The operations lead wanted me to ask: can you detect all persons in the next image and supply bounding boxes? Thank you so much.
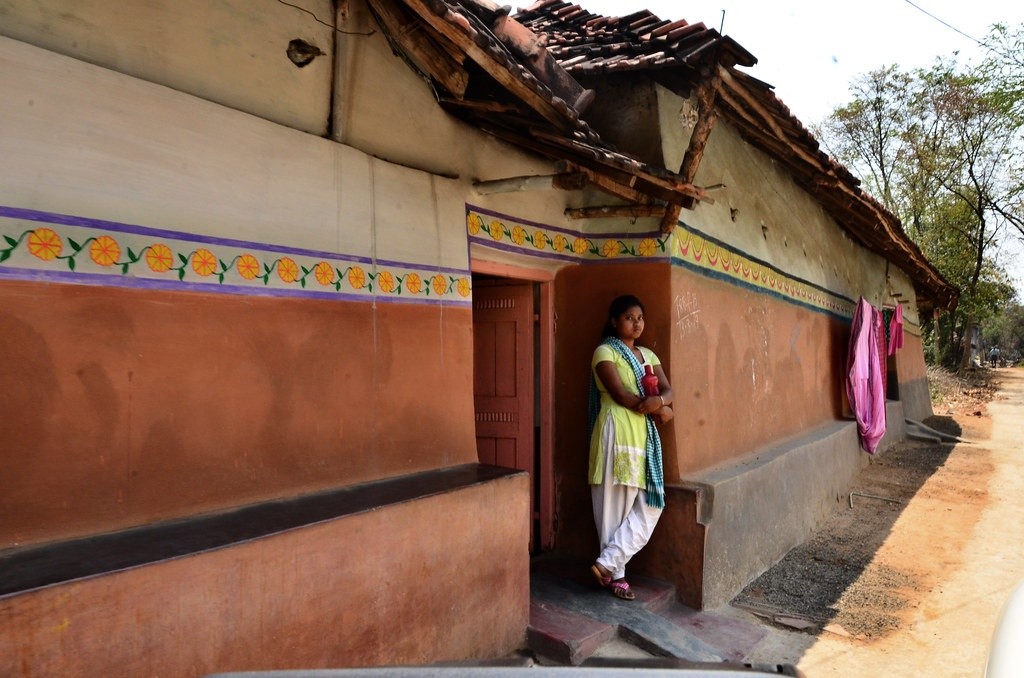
[588,293,675,600]
[987,347,1000,368]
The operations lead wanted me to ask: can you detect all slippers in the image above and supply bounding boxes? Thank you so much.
[612,582,636,600]
[591,565,610,588]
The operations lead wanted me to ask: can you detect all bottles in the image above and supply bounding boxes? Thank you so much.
[640,365,663,426]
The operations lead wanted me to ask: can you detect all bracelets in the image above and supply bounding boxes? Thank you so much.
[660,395,665,406]
